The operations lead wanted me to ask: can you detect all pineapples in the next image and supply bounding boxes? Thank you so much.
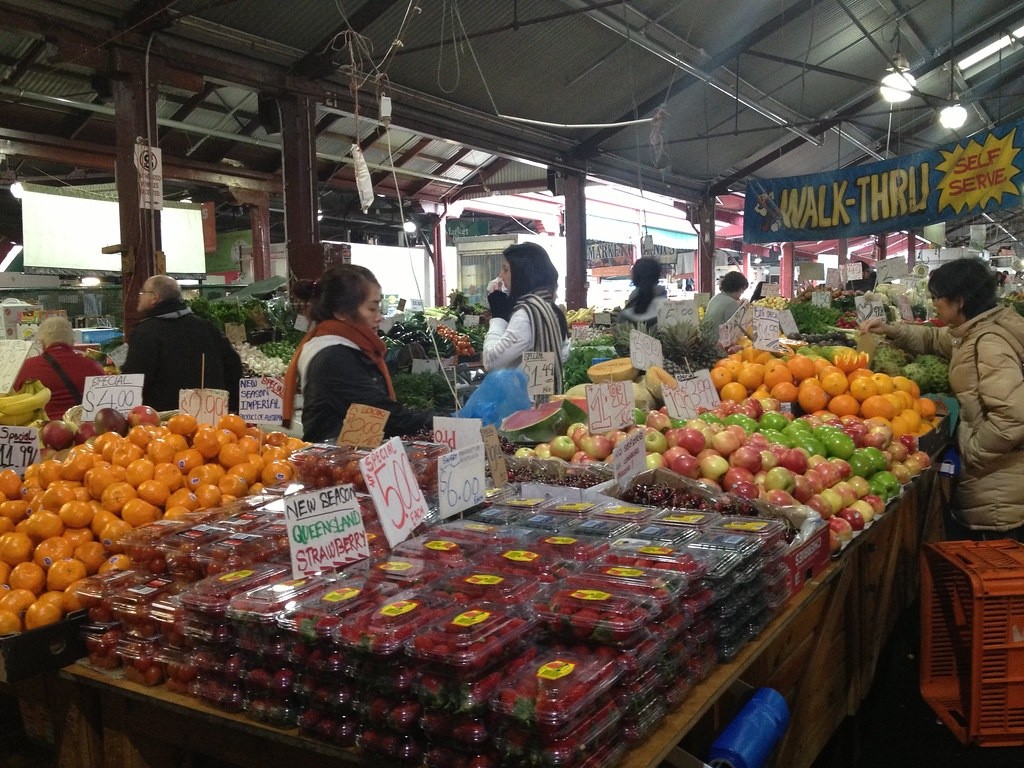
[611,321,728,377]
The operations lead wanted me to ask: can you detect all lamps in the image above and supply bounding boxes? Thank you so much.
[880,21,916,102]
[939,1,967,128]
[870,238,876,259]
[563,0,897,161]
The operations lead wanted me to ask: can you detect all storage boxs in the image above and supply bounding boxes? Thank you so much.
[920,539,1024,747]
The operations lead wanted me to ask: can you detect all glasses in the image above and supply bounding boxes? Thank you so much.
[139,289,153,295]
[931,296,953,302]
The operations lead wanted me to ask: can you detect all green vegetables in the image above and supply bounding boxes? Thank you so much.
[182,297,307,348]
[387,368,452,409]
[784,301,841,334]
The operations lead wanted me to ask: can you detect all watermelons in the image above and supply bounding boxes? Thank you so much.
[570,399,590,414]
[501,408,570,443]
[538,399,589,422]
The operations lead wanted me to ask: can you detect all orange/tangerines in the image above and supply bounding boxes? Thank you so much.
[0,413,314,634]
[708,345,943,440]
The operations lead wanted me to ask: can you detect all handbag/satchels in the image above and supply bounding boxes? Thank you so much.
[449,369,532,429]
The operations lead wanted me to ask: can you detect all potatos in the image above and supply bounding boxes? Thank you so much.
[788,285,864,306]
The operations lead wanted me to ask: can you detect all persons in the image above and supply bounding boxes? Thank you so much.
[858,255,1024,543]
[120,274,243,416]
[1014,260,1024,278]
[622,255,668,315]
[749,281,767,304]
[997,270,1011,287]
[13,317,106,421]
[297,263,433,443]
[701,270,748,327]
[841,260,874,295]
[482,240,568,395]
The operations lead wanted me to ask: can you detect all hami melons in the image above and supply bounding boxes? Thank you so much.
[567,356,679,411]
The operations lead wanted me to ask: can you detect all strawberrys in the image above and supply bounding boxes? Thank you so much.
[74,451,714,768]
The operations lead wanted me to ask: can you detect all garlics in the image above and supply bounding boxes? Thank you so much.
[231,341,289,376]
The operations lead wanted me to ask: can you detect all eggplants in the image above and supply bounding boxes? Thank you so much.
[377,324,432,350]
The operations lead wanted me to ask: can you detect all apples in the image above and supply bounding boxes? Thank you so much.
[512,398,929,553]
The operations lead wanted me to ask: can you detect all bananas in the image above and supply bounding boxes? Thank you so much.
[0,378,77,448]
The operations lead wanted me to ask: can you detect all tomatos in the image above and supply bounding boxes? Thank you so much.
[436,325,475,356]
